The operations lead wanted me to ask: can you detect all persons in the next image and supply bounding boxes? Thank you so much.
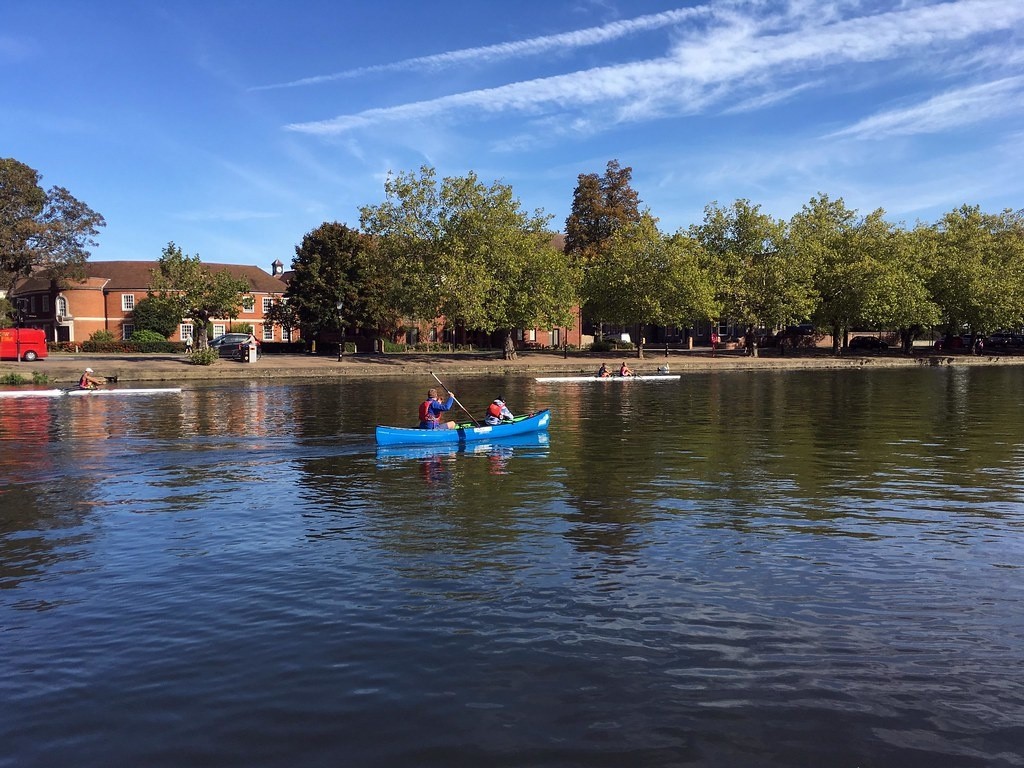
[185,333,193,354]
[598,363,609,377]
[242,332,255,354]
[486,395,514,426]
[418,389,456,430]
[79,368,98,388]
[620,362,632,376]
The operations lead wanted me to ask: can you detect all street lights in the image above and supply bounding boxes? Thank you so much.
[335,299,344,361]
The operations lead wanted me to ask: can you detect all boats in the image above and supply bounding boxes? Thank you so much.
[2,388,182,395]
[375,430,550,469]
[534,375,681,382]
[375,408,550,447]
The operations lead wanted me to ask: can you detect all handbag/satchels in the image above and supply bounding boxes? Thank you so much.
[185,341,186,345]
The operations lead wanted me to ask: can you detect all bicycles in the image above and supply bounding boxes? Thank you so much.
[232,343,262,362]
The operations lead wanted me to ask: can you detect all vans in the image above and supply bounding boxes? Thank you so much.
[0,325,49,360]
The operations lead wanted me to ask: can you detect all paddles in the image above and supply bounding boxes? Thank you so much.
[430,370,483,427]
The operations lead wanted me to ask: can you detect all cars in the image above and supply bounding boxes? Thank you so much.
[849,335,888,349]
[934,334,1024,349]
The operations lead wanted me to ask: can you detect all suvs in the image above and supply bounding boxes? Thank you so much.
[208,334,261,358]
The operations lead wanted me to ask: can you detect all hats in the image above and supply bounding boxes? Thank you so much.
[622,362,626,365]
[248,331,251,333]
[85,368,93,372]
[498,396,506,401]
[602,363,606,365]
